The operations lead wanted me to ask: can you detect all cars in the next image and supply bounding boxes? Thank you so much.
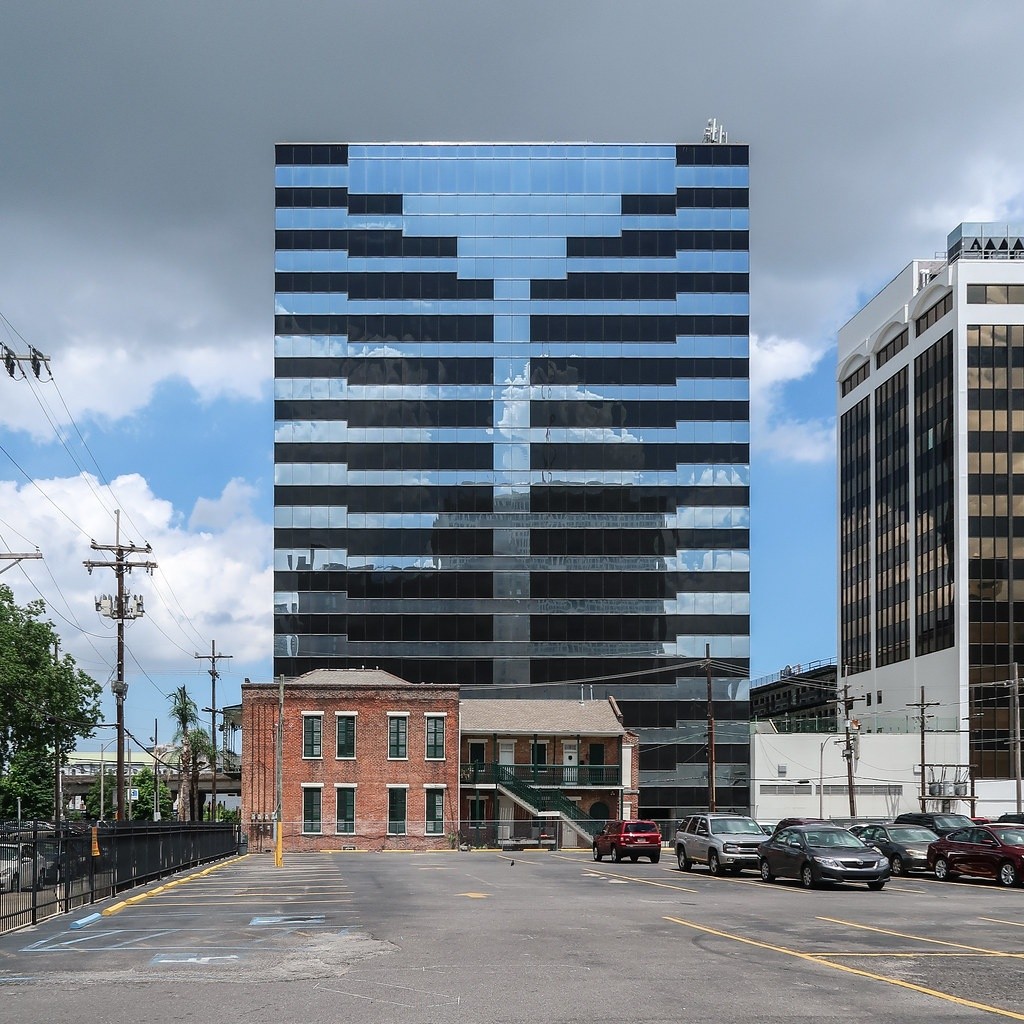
[755,824,892,891]
[855,823,941,876]
[0,819,112,894]
[926,822,1024,887]
[749,821,776,837]
[969,811,1024,827]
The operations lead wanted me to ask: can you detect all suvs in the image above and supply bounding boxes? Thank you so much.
[772,817,845,844]
[674,811,772,877]
[592,818,663,864]
[894,812,978,838]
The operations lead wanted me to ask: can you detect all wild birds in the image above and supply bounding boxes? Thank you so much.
[511,860,514,866]
